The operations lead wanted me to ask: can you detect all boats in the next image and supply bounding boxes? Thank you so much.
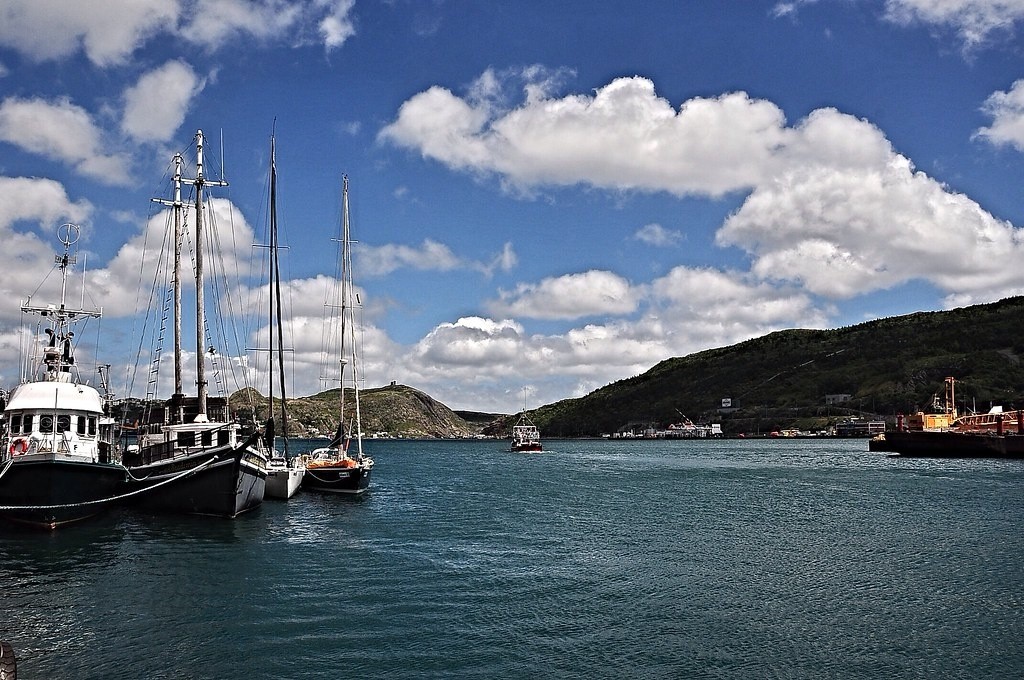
[510,413,542,453]
[0,220,124,527]
[884,377,1024,456]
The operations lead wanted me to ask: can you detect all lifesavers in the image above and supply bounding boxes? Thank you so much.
[10,439,27,457]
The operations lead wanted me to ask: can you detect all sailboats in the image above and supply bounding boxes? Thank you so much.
[128,131,266,519]
[306,173,374,494]
[247,117,307,498]
